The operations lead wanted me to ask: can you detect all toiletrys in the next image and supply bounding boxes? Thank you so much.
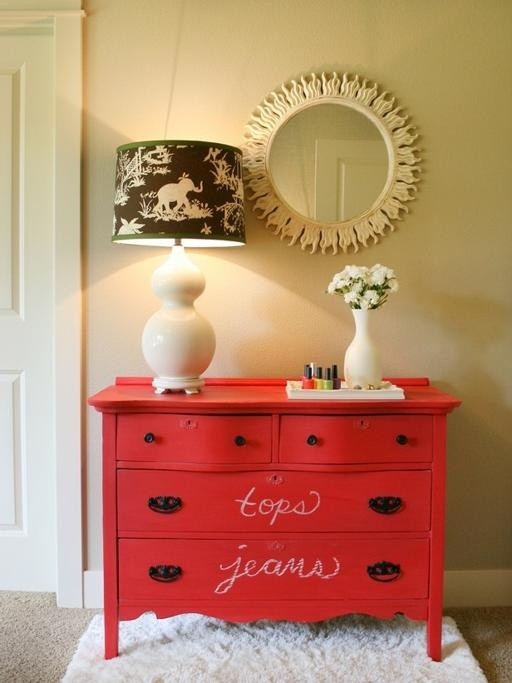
[300,359,343,391]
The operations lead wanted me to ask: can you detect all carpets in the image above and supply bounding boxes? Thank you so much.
[60,610,488,683]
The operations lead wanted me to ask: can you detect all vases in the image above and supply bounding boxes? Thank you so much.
[344,308,379,391]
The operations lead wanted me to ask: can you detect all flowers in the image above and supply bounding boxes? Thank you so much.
[325,262,398,310]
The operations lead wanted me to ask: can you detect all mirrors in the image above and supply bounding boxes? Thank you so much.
[240,71,422,256]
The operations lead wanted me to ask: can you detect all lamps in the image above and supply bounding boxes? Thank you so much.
[111,140,248,394]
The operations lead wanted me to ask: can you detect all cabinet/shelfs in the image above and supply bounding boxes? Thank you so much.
[102,409,447,662]
